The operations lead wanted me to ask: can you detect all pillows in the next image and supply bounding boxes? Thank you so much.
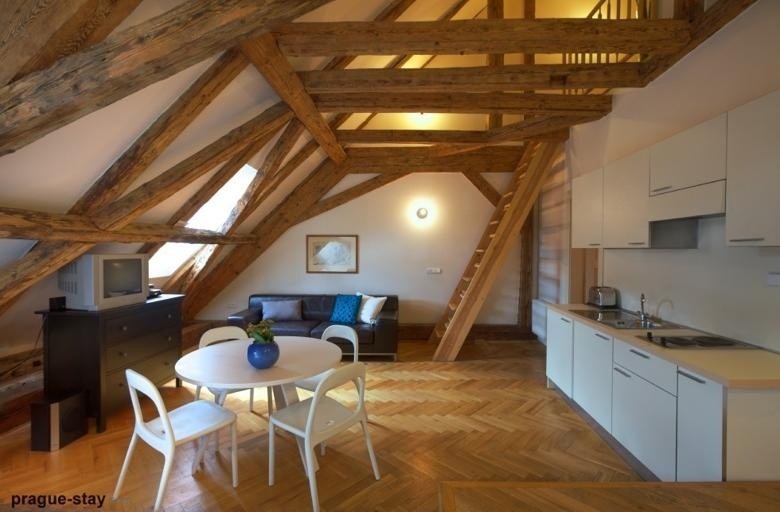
[356,292,386,323]
[261,300,302,320]
[331,293,362,324]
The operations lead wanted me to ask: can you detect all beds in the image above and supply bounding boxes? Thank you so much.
[174,336,342,473]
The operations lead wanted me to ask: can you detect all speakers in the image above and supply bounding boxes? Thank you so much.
[30,388,88,452]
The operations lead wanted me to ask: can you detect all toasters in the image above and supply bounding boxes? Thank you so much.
[588,285,618,309]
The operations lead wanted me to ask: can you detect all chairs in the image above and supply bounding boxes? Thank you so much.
[268,363,381,512]
[295,325,368,456]
[112,368,238,512]
[194,326,272,452]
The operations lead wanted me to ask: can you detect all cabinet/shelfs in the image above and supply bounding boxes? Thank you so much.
[570,88,780,250]
[546,307,779,483]
[34,293,187,433]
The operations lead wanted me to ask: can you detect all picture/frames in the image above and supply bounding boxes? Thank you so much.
[306,234,359,274]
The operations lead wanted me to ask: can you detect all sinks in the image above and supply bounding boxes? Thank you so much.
[598,319,663,331]
[567,306,639,320]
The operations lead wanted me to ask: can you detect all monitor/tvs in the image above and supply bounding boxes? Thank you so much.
[58,254,149,312]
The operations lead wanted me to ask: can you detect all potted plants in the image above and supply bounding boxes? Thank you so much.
[247,319,280,368]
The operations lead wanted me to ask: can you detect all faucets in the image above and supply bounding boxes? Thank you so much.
[640,293,648,327]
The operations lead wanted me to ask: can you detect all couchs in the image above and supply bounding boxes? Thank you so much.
[227,294,400,362]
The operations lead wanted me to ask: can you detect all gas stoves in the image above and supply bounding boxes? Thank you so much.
[634,332,763,349]
